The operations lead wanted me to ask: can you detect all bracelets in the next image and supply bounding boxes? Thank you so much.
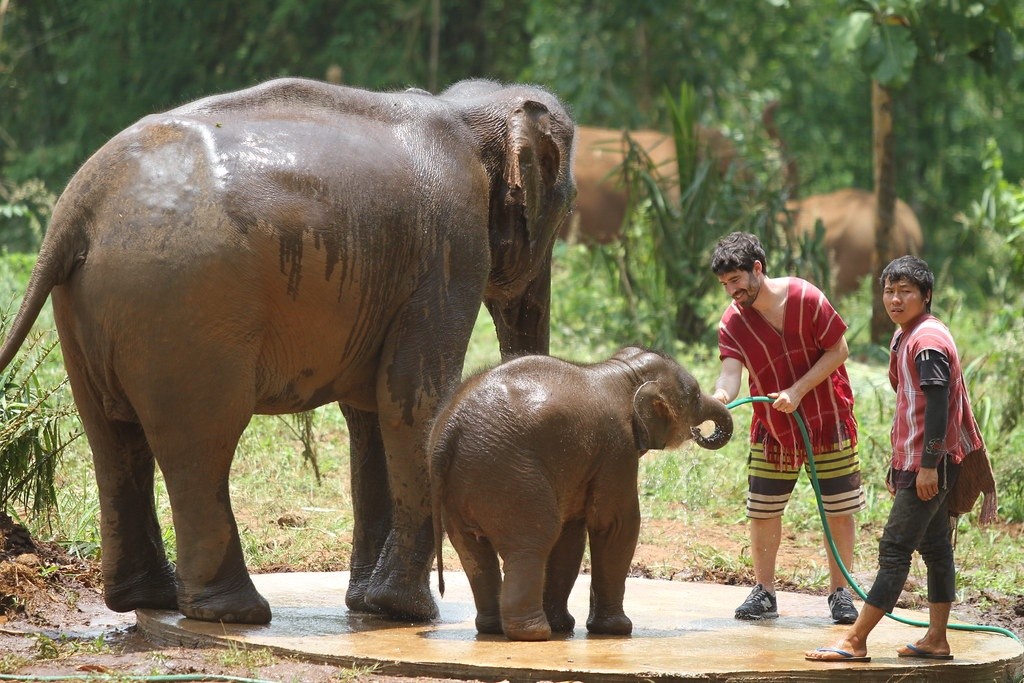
[715,389,728,406]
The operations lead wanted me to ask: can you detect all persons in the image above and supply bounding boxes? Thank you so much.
[803,254,980,662]
[712,231,863,624]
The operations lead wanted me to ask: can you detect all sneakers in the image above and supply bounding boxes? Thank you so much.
[735,583,779,619]
[828,587,859,622]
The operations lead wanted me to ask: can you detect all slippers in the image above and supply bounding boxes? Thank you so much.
[805,645,870,662]
[899,643,955,660]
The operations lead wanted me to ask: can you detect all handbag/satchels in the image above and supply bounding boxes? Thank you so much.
[884,415,998,526]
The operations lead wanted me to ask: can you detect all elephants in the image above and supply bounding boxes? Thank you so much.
[422,344,733,642]
[0,77,578,625]
[554,101,924,309]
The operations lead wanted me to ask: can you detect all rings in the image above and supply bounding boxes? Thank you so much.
[785,408,788,414]
